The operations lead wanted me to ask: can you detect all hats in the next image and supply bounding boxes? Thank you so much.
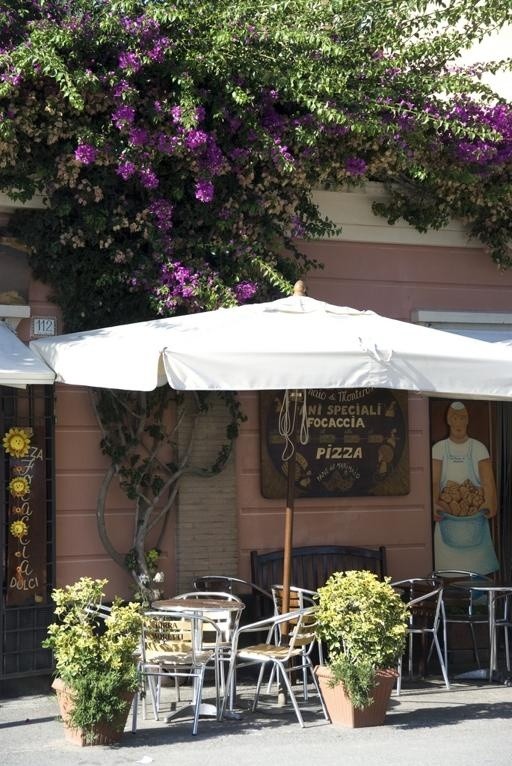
[451,402,465,411]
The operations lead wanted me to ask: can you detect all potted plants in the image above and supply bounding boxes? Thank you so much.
[39,575,169,746]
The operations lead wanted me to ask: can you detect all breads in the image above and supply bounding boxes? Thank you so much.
[436,479,484,516]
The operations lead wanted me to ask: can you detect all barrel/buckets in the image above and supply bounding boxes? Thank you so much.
[436,509,486,549]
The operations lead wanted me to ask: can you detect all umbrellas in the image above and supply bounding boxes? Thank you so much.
[29,276,511,707]
